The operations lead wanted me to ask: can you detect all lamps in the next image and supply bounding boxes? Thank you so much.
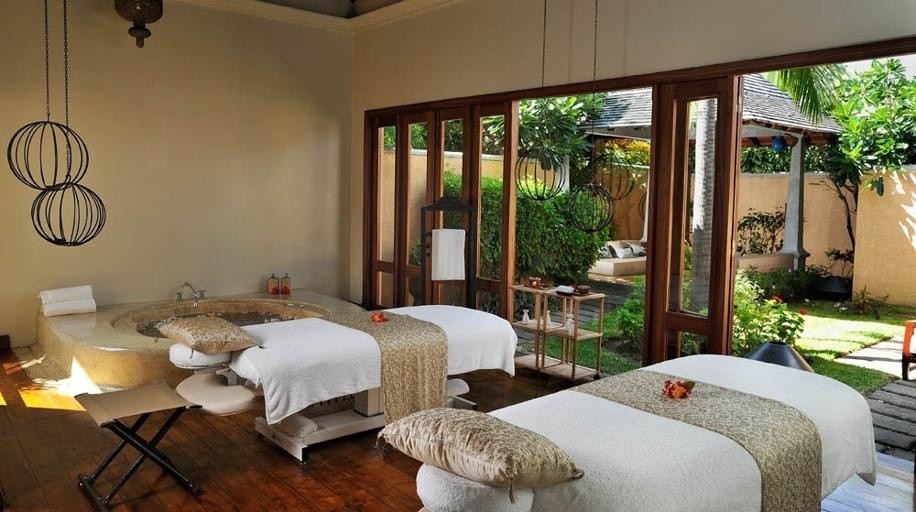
[902,318,916,382]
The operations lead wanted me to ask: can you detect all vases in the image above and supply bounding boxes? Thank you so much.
[368,311,390,323]
[660,378,697,401]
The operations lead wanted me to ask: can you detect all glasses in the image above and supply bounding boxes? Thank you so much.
[113,0,163,49]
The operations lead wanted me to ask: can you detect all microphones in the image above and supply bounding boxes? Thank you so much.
[74,378,206,512]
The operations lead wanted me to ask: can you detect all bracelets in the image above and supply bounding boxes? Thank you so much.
[574,285,592,294]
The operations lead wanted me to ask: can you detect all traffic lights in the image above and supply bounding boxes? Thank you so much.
[157,314,260,354]
[375,404,583,487]
[626,241,647,257]
[613,246,633,260]
[594,244,611,260]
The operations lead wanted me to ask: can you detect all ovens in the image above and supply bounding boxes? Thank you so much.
[163,302,521,461]
[413,352,876,512]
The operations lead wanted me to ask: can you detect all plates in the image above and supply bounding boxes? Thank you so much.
[543,288,605,386]
[507,281,565,375]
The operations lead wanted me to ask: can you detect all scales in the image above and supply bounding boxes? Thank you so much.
[35,286,370,391]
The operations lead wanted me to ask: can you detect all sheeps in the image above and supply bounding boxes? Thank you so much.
[185,281,201,310]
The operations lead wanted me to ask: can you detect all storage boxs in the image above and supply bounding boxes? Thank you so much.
[587,237,649,276]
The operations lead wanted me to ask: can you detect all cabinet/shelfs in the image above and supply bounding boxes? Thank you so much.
[268,271,291,295]
[520,307,575,336]
